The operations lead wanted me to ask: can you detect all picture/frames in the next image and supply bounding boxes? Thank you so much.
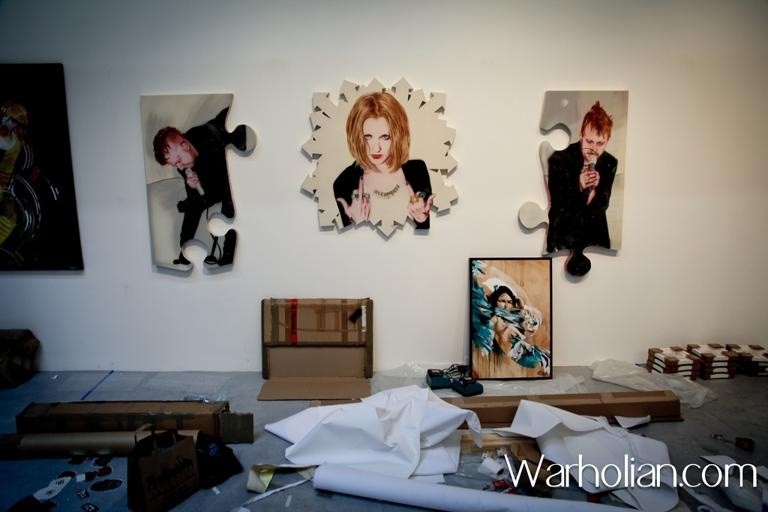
[468,257,552,380]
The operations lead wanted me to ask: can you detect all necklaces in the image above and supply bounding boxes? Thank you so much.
[371,182,401,200]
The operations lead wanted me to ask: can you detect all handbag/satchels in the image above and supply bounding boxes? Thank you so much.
[127,423,200,511]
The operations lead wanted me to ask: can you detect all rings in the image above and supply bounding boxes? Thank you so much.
[417,191,428,199]
[350,193,359,201]
[409,196,418,204]
[362,191,370,199]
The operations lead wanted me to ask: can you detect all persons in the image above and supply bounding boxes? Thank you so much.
[546,99,618,276]
[472,261,550,375]
[332,91,436,230]
[152,106,247,267]
